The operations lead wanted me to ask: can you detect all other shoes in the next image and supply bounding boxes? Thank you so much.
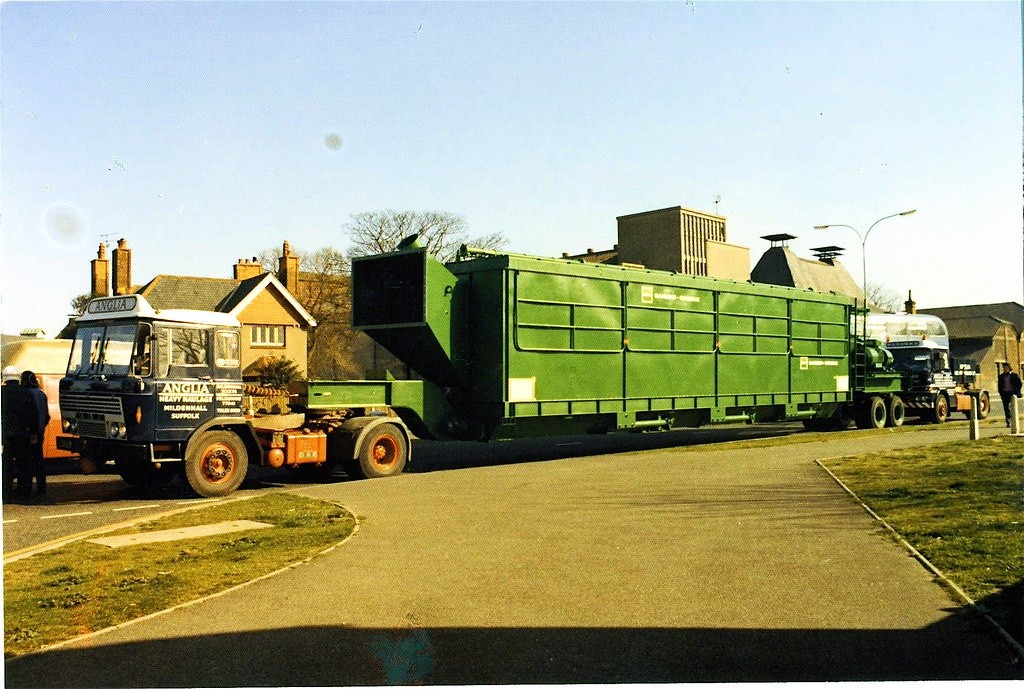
[13,495,27,499]
[1007,423,1011,428]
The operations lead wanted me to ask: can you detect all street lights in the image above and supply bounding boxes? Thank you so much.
[814,208,917,309]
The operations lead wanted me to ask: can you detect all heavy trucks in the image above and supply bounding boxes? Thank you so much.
[56,232,906,499]
[881,334,991,423]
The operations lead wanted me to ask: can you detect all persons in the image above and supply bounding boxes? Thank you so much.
[1,366,39,503]
[998,362,1022,427]
[20,370,51,505]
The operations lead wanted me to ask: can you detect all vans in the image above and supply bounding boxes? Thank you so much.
[1,338,151,475]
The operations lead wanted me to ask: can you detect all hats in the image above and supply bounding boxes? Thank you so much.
[5,380,19,383]
